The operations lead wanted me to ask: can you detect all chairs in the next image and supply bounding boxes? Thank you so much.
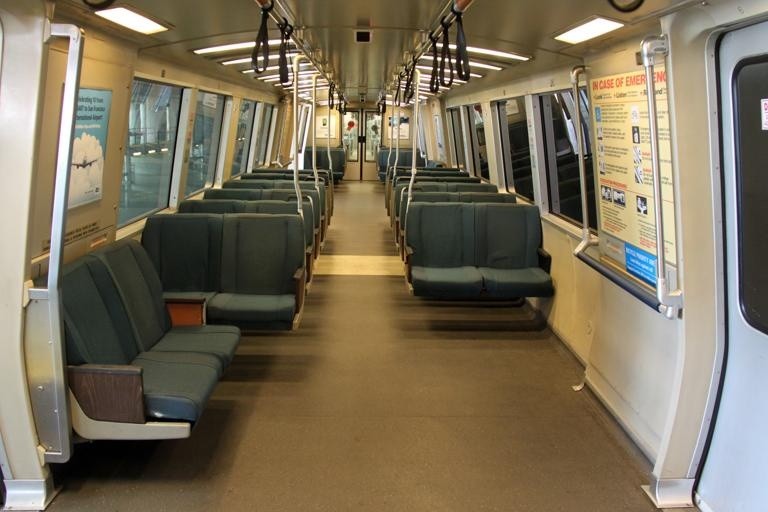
[377,144,557,303]
[138,144,348,335]
[27,252,222,444]
[87,236,242,370]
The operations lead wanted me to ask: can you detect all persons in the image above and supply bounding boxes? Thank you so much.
[341,120,355,160]
[369,124,380,162]
[635,195,648,213]
